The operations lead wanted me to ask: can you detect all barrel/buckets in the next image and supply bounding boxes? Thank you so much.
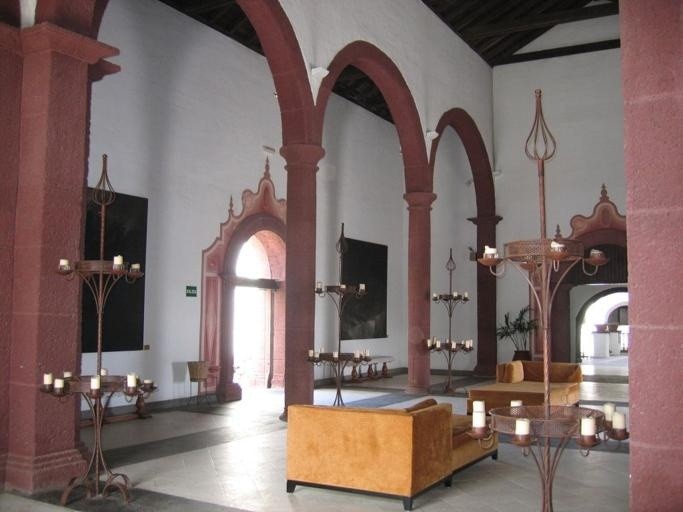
[187,361,210,381]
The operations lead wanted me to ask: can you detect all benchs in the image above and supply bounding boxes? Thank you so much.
[464,357,582,417]
[283,398,500,510]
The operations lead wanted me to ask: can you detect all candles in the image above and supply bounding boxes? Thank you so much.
[426,335,472,349]
[315,280,366,291]
[307,346,370,359]
[59,254,140,272]
[432,290,468,300]
[482,240,603,257]
[39,368,155,391]
[471,398,628,437]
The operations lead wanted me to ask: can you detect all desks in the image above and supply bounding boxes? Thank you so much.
[348,355,394,381]
